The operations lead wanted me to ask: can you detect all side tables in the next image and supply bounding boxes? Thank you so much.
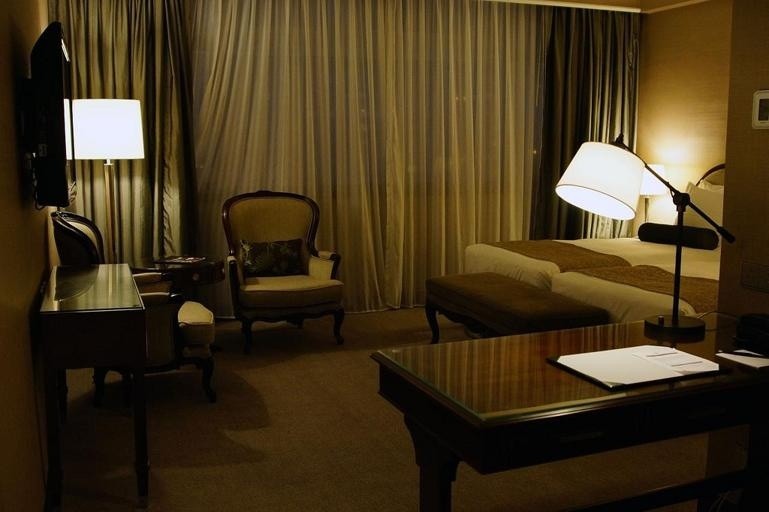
[126,255,226,300]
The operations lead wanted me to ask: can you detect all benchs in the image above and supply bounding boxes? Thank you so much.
[424,271,608,347]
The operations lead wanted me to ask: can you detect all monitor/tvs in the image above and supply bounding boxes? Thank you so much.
[16,20,69,207]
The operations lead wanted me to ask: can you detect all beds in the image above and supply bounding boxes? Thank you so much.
[463,237,726,293]
[552,248,721,323]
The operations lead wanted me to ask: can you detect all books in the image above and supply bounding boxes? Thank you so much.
[545,343,720,390]
[151,255,207,265]
[715,349,769,371]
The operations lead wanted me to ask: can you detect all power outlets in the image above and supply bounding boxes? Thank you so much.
[742,260,769,295]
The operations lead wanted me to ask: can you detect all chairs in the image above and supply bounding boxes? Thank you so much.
[222,190,345,356]
[51,211,219,409]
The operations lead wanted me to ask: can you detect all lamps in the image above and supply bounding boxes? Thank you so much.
[638,165,666,225]
[71,98,145,262]
[554,133,736,337]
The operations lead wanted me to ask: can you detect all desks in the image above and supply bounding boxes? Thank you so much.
[29,263,149,512]
[368,313,769,512]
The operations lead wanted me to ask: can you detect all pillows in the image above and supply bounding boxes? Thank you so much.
[678,182,724,247]
[237,238,308,277]
[637,223,719,250]
